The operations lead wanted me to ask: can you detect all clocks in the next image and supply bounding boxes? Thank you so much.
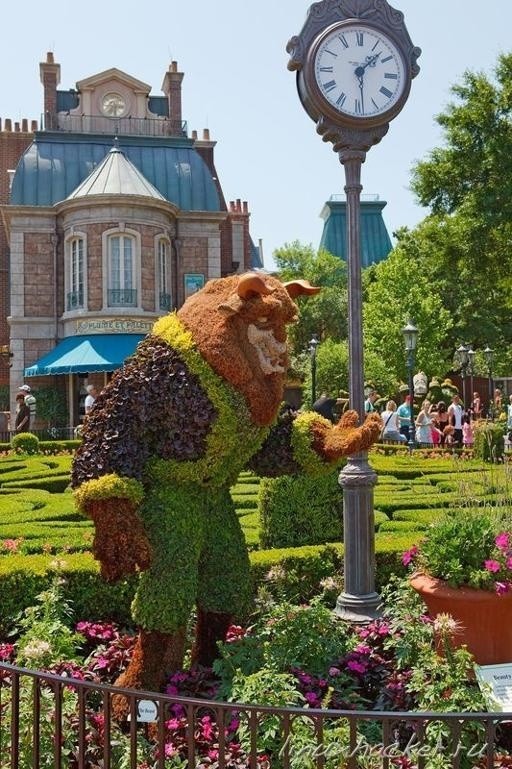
[283,0,422,164]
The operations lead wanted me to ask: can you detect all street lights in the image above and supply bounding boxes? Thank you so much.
[401,321,420,450]
[456,344,495,411]
[308,333,320,404]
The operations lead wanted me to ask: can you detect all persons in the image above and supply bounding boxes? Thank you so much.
[15,384,37,432]
[14,393,30,434]
[84,384,98,414]
[283,386,512,449]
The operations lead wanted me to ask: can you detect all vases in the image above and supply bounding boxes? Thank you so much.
[408,570,511,666]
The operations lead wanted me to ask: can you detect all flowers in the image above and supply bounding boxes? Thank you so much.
[391,512,510,596]
